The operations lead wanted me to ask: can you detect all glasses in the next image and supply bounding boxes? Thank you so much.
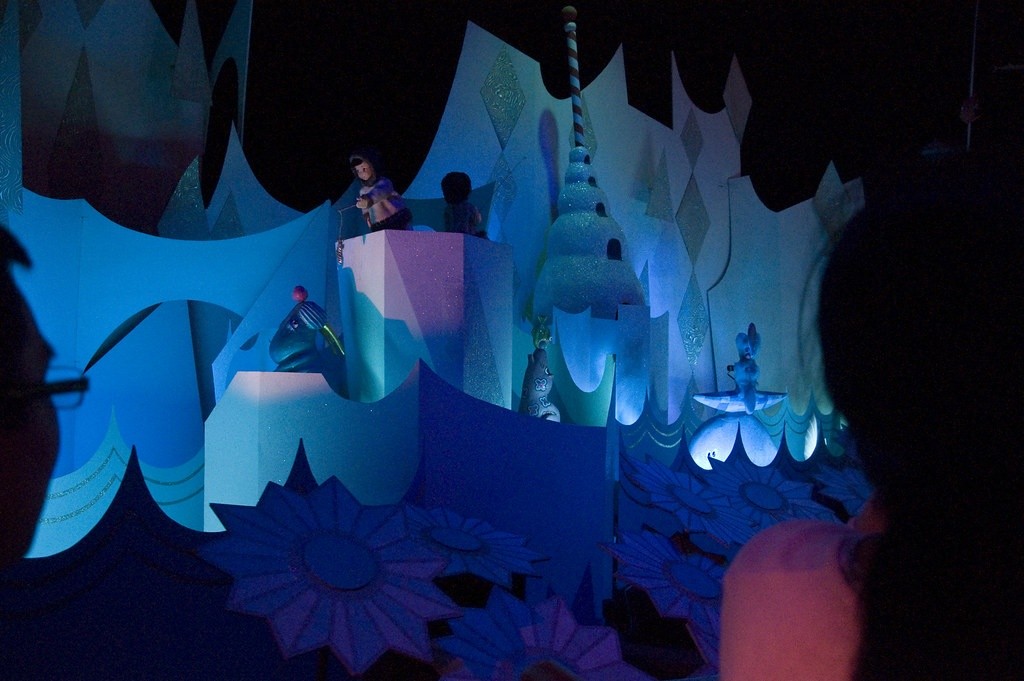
[0,363,90,408]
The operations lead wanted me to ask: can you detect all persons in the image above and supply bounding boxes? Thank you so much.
[0,218,93,681]
[441,170,491,241]
[348,148,412,233]
[717,185,1024,681]
